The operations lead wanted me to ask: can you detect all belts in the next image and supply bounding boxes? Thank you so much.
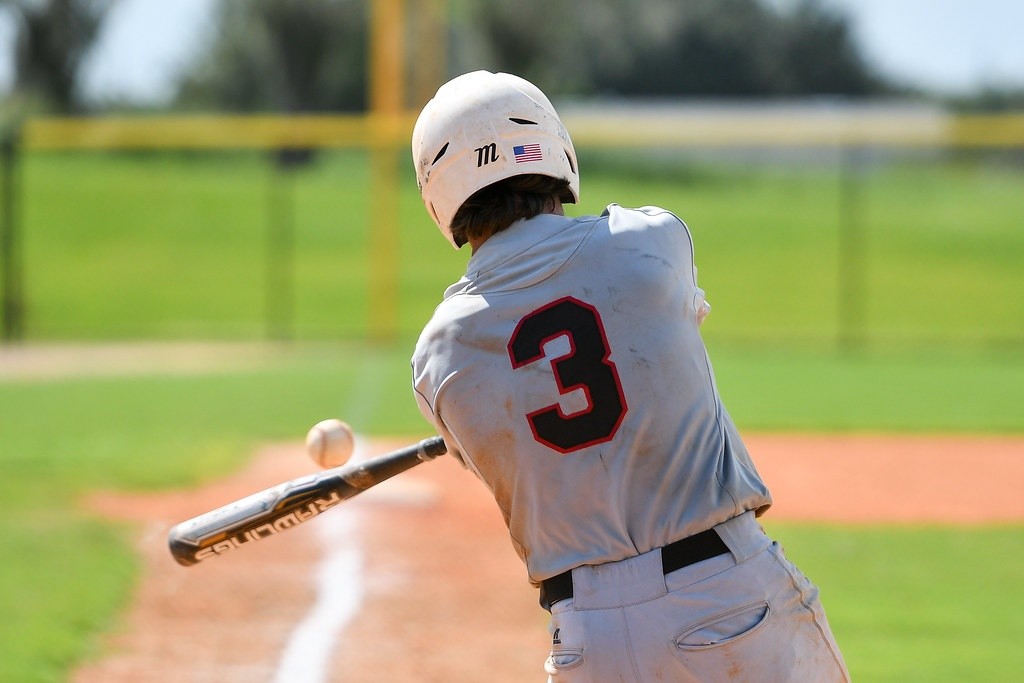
[540,529,731,614]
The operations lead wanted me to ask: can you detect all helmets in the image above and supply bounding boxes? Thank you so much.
[412,70,579,252]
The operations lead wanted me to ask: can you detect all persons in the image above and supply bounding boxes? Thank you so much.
[410,67,853,683]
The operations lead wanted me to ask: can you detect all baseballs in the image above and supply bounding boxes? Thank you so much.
[308,417,357,470]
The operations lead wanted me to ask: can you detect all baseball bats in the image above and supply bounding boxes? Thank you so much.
[167,438,448,570]
